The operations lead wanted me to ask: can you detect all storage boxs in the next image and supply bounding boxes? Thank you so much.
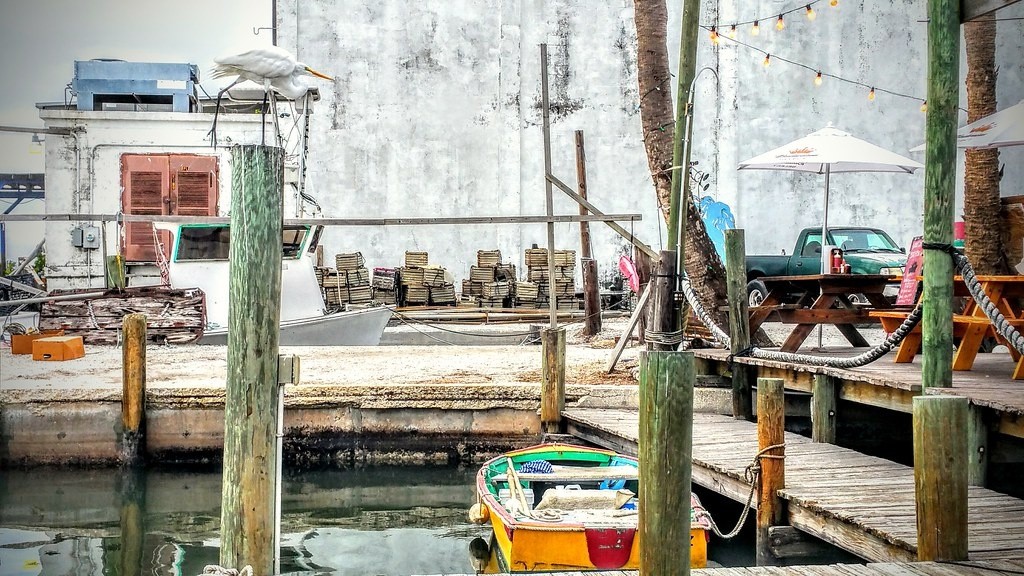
[11,329,65,355]
[32,336,86,361]
[314,247,579,311]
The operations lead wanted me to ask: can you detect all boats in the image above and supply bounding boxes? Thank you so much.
[152,218,398,345]
[469,441,710,573]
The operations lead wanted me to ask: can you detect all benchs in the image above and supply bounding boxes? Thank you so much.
[720,303,1024,380]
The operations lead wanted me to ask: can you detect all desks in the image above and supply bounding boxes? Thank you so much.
[749,274,896,354]
[896,275,1024,370]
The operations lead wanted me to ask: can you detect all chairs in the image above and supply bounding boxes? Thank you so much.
[840,240,856,249]
[803,242,822,257]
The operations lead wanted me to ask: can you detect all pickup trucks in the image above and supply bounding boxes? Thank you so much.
[743,226,909,309]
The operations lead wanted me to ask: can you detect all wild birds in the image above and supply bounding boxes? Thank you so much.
[205,48,333,150]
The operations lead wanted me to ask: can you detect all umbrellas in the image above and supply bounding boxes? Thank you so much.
[908,99,1024,152]
[736,122,926,347]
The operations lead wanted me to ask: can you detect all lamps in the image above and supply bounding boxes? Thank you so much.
[699,0,927,114]
